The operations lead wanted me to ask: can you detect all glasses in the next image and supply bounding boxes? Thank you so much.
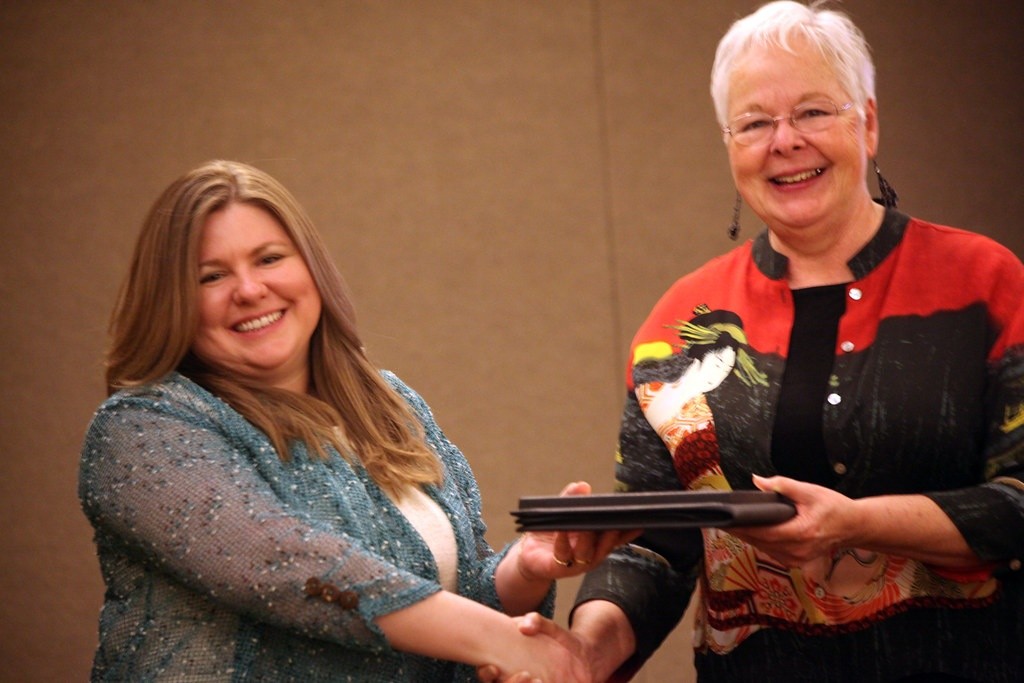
[722,98,856,146]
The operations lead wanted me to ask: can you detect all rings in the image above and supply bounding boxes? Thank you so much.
[554,554,575,566]
[573,559,595,564]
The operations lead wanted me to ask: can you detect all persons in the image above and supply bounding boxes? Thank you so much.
[476,0,1024,682]
[78,162,643,682]
